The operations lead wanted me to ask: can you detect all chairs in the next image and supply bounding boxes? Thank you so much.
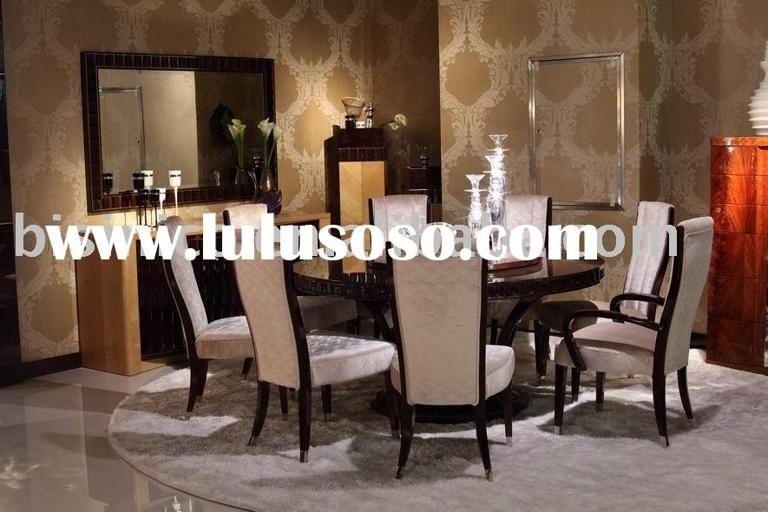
[155,216,288,417]
[489,195,551,380]
[386,239,515,483]
[367,194,432,339]
[222,203,359,334]
[231,242,398,463]
[555,216,713,448]
[532,200,675,399]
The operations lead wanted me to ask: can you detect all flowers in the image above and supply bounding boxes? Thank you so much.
[257,118,283,167]
[228,118,247,167]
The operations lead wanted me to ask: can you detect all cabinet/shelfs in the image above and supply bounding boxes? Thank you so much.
[705,135,767,375]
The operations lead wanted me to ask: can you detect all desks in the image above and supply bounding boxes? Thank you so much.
[76,213,331,375]
[293,255,605,425]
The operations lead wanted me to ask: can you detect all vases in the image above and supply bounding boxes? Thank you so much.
[259,168,275,195]
[745,61,768,134]
[235,167,249,187]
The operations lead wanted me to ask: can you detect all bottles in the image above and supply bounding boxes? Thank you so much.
[344,113,356,129]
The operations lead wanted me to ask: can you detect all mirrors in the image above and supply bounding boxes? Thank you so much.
[81,51,278,213]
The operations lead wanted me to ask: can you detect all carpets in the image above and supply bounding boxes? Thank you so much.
[107,348,768,512]
